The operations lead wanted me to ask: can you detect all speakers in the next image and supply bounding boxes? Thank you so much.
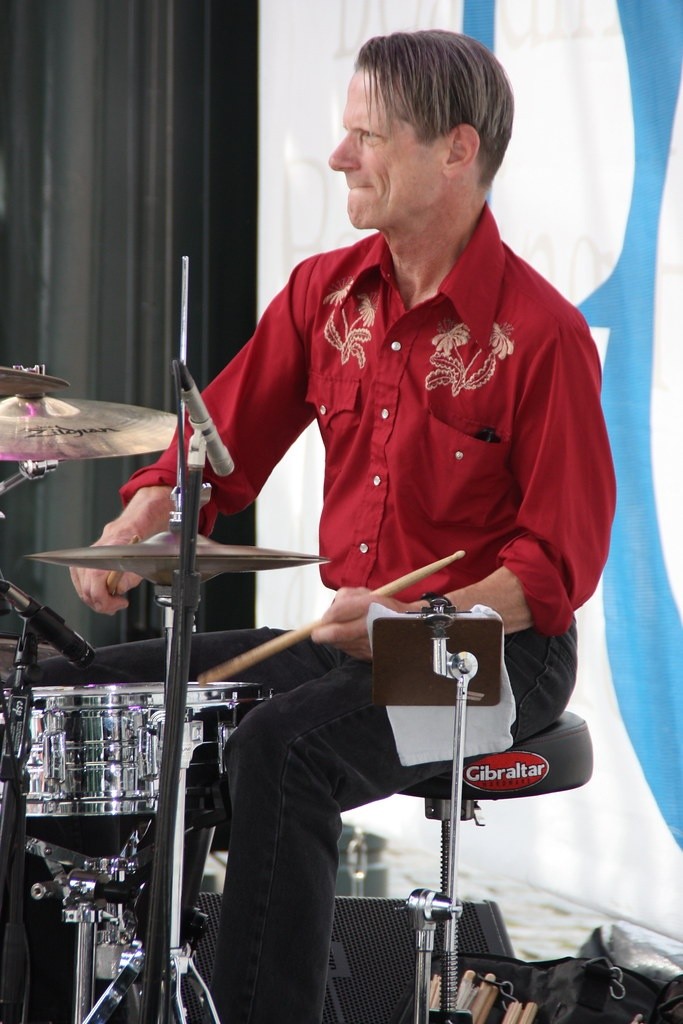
[181,892,517,1024]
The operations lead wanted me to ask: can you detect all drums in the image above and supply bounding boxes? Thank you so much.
[2,675,270,817]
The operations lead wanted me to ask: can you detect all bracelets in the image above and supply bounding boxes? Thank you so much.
[420,593,452,608]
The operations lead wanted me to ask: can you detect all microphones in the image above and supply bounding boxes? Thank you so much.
[176,361,234,476]
[0,579,97,672]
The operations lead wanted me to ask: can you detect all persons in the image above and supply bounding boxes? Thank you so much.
[6,28,618,1024]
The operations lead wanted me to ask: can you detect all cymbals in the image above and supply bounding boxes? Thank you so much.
[0,362,70,403]
[0,393,182,461]
[21,530,336,601]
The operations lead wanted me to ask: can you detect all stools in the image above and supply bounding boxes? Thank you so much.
[388,711,593,1024]
